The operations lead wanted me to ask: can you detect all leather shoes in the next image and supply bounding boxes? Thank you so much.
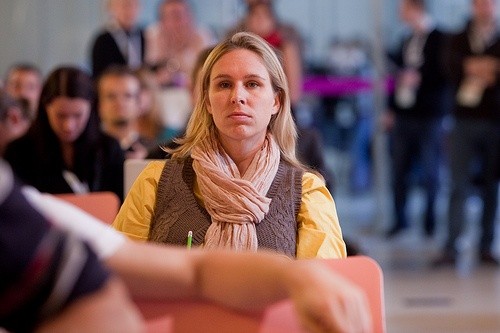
[387,214,500,270]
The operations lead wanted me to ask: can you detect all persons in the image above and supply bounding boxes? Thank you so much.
[0,0,305,161]
[386,0,500,269]
[3,67,124,203]
[0,159,371,333]
[111,32,347,260]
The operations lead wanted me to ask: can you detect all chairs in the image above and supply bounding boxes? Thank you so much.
[59,192,118,233]
[128,257,385,332]
